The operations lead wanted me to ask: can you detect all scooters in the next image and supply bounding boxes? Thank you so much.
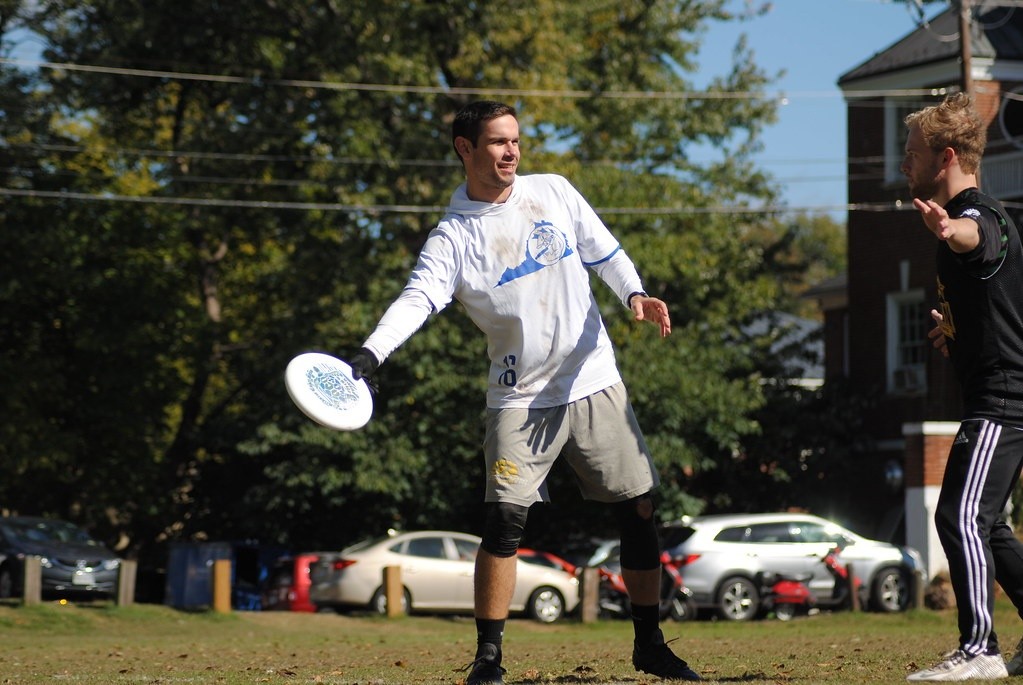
[755,534,865,620]
[594,550,701,623]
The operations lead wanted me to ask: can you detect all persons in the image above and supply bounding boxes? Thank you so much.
[343,100,705,685]
[898,92,1023,681]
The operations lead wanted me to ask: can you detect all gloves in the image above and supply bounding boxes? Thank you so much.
[344,347,379,389]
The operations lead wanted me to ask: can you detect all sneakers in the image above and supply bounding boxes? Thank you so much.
[1007,637,1023,675]
[907,649,1010,682]
[632,629,698,680]
[461,642,506,685]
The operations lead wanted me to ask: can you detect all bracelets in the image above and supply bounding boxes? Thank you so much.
[628,292,648,310]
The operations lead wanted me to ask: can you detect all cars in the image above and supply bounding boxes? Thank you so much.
[460,545,580,578]
[671,510,929,620]
[0,514,120,602]
[308,527,581,623]
[583,538,629,573]
[257,550,324,613]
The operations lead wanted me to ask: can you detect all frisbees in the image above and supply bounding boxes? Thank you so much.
[284,353,373,431]
